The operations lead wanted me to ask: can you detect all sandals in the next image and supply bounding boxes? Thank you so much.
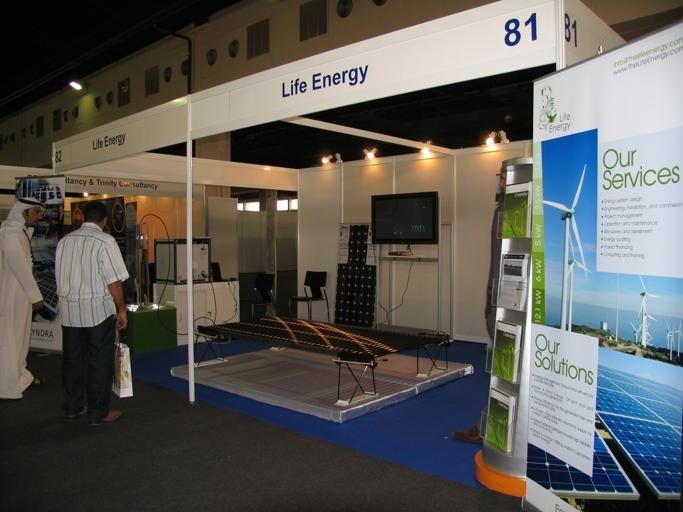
[31,377,47,385]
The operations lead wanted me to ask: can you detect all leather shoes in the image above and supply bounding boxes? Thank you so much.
[454,427,483,442]
[64,406,121,422]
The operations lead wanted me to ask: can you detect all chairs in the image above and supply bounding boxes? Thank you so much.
[289,271,331,322]
[251,272,274,319]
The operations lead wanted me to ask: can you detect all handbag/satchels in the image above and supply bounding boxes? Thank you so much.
[112,342,134,398]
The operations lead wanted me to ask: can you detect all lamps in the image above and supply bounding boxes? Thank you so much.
[321,153,343,165]
[485,130,509,144]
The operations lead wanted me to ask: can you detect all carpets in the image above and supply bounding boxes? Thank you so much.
[129,340,492,489]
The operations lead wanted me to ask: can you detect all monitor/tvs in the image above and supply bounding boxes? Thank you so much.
[371,190,438,258]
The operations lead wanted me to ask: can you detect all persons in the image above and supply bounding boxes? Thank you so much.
[0,197,44,400]
[55,202,130,424]
